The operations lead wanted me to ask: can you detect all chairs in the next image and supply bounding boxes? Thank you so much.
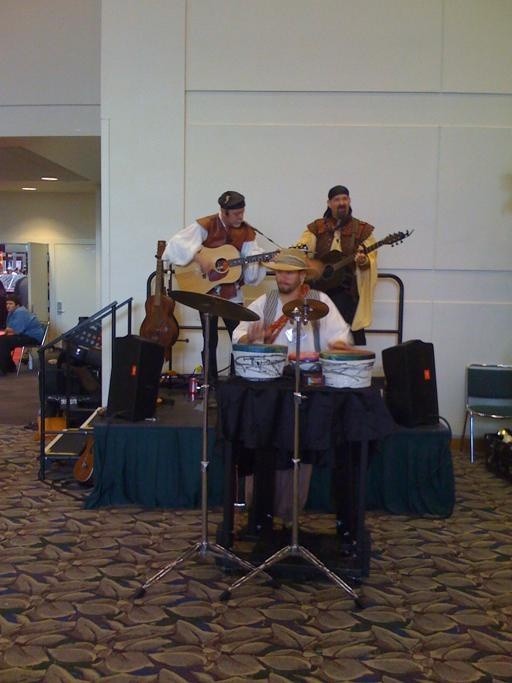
[11,324,50,376]
[459,363,511,464]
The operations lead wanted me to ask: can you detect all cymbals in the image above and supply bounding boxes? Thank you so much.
[168,290,261,321]
[282,298,329,320]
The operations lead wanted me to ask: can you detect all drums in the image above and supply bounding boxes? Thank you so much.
[319,349,375,389]
[233,344,288,382]
[289,351,320,371]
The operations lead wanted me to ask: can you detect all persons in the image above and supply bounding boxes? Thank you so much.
[3,267,18,275]
[161,191,267,410]
[0,295,46,376]
[296,185,378,347]
[232,248,355,552]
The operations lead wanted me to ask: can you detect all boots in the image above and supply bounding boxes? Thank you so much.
[201,351,218,408]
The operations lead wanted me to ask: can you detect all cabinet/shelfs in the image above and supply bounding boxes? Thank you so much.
[0,242,50,324]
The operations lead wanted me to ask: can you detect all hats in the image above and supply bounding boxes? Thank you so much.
[218,191,245,209]
[261,249,319,279]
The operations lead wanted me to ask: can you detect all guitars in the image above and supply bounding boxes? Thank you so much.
[309,230,413,292]
[175,244,308,293]
[73,435,95,486]
[140,240,179,351]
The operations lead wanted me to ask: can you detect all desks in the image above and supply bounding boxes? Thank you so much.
[221,377,383,585]
